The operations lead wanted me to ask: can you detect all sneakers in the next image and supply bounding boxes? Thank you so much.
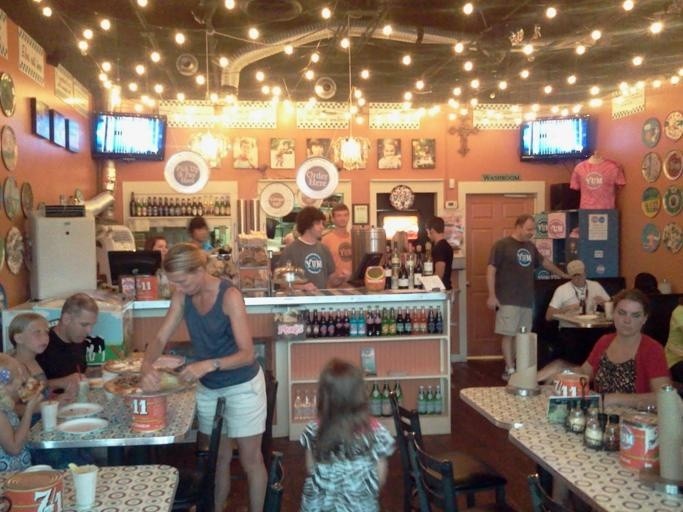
[501,367,515,381]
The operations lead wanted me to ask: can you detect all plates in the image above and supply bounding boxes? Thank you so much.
[640,111,682,253]
[1,71,34,274]
[390,185,415,210]
[104,375,196,397]
[59,402,104,418]
[104,352,187,374]
[57,418,109,433]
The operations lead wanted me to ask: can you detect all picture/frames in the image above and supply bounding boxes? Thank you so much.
[662,150,682,180]
[664,110,683,141]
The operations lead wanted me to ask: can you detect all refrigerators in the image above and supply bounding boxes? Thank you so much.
[33,290,134,365]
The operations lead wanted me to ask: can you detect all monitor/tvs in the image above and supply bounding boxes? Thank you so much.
[94,111,166,163]
[519,112,589,162]
[106,249,162,286]
[380,210,421,241]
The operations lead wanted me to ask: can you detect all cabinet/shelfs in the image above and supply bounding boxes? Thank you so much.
[287,300,452,441]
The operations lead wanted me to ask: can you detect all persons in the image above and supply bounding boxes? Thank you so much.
[144,236,169,257]
[484,214,572,382]
[544,258,683,506]
[274,144,292,167]
[1,291,110,486]
[378,139,401,169]
[424,215,454,291]
[233,139,257,169]
[272,202,354,293]
[568,151,628,210]
[418,145,434,165]
[296,355,397,511]
[310,139,324,157]
[188,216,223,257]
[139,240,268,510]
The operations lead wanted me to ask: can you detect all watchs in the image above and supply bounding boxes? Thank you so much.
[213,359,221,372]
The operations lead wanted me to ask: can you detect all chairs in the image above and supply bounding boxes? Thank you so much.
[388,392,508,512]
[229,370,279,481]
[172,397,225,511]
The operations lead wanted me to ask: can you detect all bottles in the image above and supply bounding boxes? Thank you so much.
[383,239,434,290]
[579,297,585,315]
[130,192,231,216]
[283,259,296,296]
[567,398,620,451]
[299,306,443,337]
[294,380,441,419]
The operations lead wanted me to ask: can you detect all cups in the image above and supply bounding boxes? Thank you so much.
[76,381,90,403]
[604,302,614,320]
[72,465,100,508]
[40,400,60,431]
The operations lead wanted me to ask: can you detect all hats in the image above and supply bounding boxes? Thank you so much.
[567,260,585,276]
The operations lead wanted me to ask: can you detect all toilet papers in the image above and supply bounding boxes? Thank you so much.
[507,332,536,390]
[656,389,682,482]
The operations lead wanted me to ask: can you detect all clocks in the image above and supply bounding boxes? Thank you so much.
[641,152,662,182]
